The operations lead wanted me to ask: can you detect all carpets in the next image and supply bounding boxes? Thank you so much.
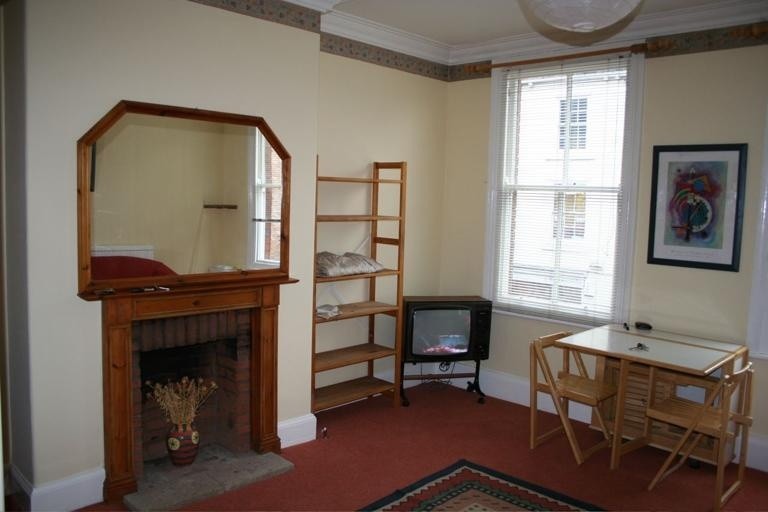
[356,459,610,512]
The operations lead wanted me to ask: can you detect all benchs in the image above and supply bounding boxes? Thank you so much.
[530,331,617,466]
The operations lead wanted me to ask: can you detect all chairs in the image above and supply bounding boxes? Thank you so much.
[646,361,754,512]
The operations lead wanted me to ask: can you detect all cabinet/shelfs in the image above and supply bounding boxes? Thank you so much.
[312,154,407,415]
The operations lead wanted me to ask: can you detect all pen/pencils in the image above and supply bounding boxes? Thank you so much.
[624,323,629,331]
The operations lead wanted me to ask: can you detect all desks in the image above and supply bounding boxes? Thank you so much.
[401,355,486,407]
[553,323,748,473]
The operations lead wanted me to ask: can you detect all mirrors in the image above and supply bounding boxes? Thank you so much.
[77,100,291,293]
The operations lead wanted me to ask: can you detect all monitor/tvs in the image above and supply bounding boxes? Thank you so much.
[402,294,493,363]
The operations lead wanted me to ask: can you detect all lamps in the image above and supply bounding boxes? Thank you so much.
[524,0,641,33]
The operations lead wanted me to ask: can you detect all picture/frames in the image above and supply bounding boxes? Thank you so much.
[647,143,748,272]
[89,143,96,192]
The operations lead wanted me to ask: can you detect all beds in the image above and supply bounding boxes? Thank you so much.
[91,245,177,280]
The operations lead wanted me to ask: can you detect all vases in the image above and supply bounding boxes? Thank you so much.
[166,416,200,466]
